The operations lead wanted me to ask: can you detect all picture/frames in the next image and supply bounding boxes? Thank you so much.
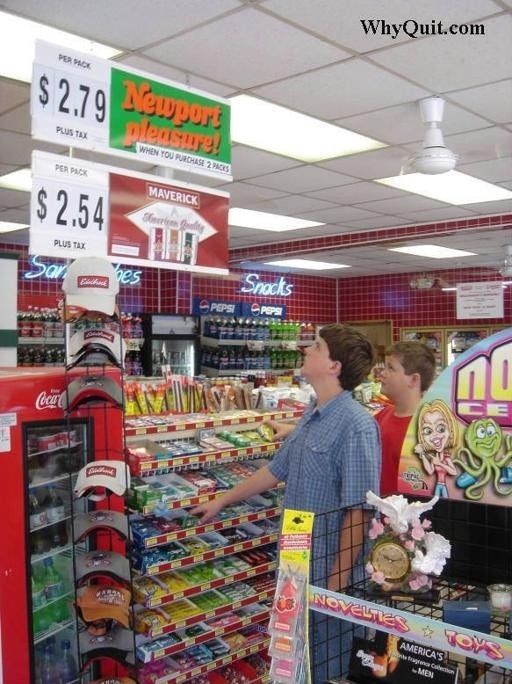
[399,323,511,375]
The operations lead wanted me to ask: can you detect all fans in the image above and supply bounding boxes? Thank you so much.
[464,231,512,277]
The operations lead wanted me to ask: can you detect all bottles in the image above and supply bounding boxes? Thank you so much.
[18,305,65,368]
[204,316,315,387]
[153,350,191,377]
[24,477,85,684]
[122,310,146,377]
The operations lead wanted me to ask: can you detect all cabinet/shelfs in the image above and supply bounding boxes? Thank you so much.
[0,313,392,684]
[306,493,512,683]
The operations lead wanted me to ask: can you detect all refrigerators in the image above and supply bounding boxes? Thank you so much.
[0,365,131,684]
[134,313,199,378]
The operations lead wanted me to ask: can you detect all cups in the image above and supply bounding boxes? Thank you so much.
[487,583,512,616]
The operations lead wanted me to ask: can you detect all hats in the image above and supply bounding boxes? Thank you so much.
[74,459,132,501]
[62,254,120,316]
[70,509,134,544]
[75,550,132,584]
[79,656,136,684]
[62,374,126,412]
[66,326,128,369]
[72,575,133,630]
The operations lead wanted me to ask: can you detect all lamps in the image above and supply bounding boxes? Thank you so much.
[407,95,457,177]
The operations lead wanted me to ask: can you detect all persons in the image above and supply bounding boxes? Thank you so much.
[262,340,436,526]
[188,322,384,684]
